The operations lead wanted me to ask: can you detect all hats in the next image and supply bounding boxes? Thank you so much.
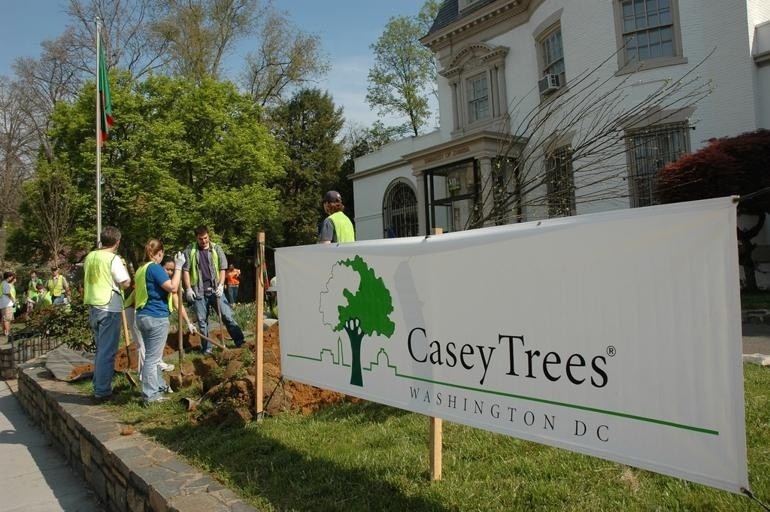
[319,190,343,203]
[51,266,57,271]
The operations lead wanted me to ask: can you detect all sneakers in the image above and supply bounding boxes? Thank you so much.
[202,346,214,356]
[90,392,113,404]
[241,343,248,349]
[144,397,171,408]
[158,387,173,395]
[162,364,175,373]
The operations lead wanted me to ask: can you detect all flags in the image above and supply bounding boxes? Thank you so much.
[97,28,112,147]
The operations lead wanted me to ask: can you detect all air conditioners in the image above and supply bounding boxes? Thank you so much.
[537,73,560,95]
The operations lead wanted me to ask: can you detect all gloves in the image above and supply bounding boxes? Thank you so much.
[215,283,224,299]
[174,251,186,272]
[186,287,196,304]
[187,323,198,335]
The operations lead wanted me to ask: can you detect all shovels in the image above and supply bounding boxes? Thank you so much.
[121,289,139,388]
[178,277,193,392]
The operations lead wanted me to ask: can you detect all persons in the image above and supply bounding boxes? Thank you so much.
[224,263,241,307]
[317,190,355,244]
[84,228,131,405]
[2,266,69,335]
[183,224,249,355]
[123,236,197,403]
